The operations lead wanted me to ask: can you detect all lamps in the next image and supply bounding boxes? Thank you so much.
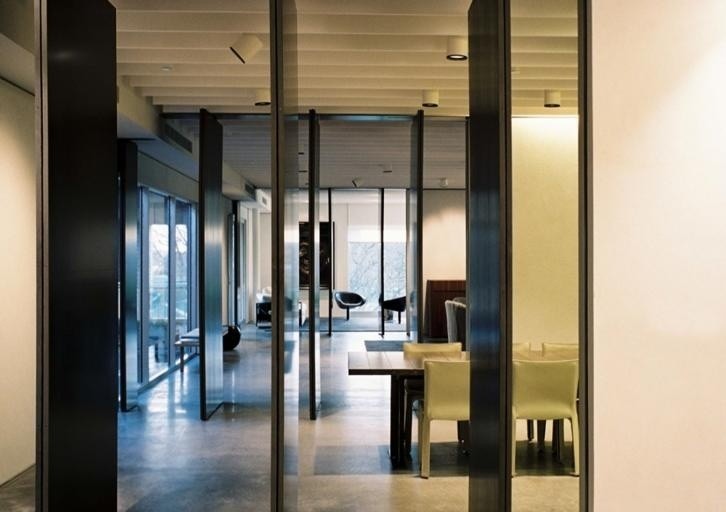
[422,90,439,107]
[447,38,468,60]
[228,33,264,64]
[544,89,561,108]
[254,88,271,105]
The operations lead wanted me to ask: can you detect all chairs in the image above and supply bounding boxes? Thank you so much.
[402,340,579,481]
[383,296,406,325]
[335,291,366,321]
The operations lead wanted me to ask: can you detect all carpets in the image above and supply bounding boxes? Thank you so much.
[264,315,406,332]
[364,340,449,351]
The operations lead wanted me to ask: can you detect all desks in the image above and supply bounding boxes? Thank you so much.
[347,349,581,472]
